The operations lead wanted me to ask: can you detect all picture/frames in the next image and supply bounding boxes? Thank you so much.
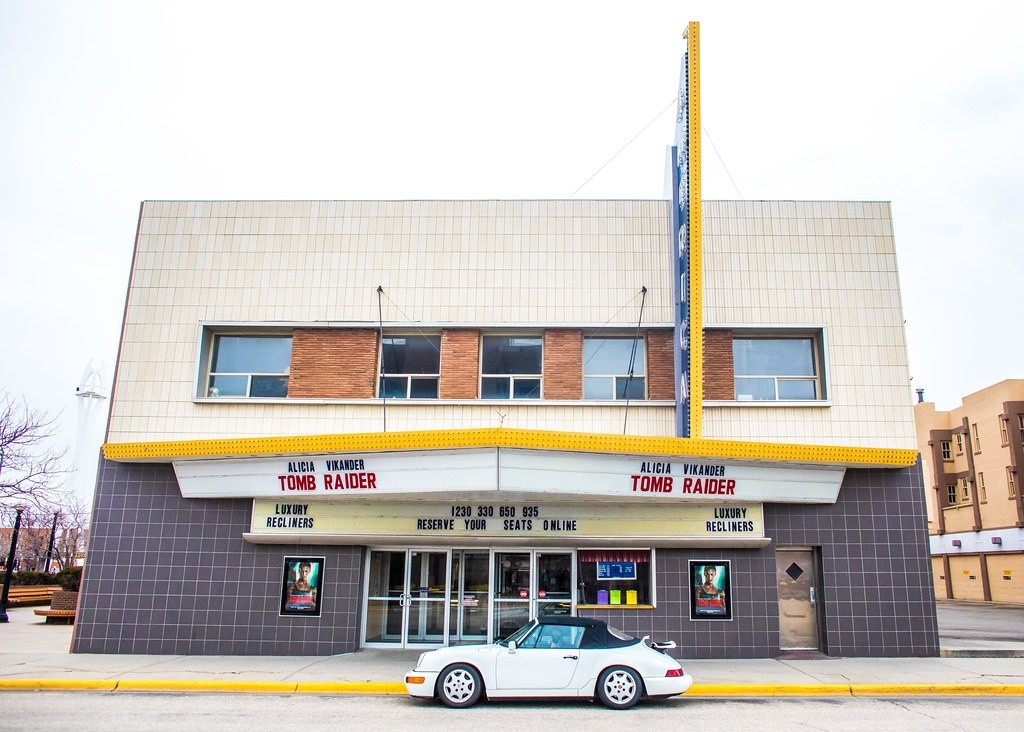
[278,555,326,618]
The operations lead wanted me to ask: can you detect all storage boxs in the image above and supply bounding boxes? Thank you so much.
[597,590,608,604]
[610,590,621,604]
[626,590,638,605]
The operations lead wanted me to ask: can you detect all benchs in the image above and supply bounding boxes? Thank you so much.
[0,584,63,609]
[34,609,76,624]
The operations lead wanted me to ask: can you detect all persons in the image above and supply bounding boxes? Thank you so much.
[695,565,727,614]
[285,561,317,610]
[551,631,562,649]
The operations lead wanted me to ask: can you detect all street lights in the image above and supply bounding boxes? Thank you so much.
[43,506,60,572]
[0,498,28,624]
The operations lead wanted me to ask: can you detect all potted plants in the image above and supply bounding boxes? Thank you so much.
[51,566,83,609]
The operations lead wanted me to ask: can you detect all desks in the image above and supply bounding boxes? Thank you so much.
[577,603,653,610]
[688,559,734,622]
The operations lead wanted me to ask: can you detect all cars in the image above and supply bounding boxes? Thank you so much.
[404,613,692,710]
[479,597,572,639]
